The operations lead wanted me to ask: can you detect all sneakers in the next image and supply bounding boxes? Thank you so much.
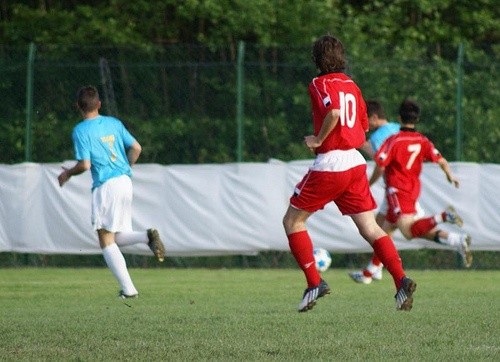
[117,290,139,299]
[457,234,472,267]
[446,205,462,226]
[395,277,417,310]
[348,271,371,284]
[298,279,331,312]
[147,228,165,262]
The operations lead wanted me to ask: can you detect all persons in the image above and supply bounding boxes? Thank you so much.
[347,100,474,284]
[282,35,417,312]
[57,86,166,299]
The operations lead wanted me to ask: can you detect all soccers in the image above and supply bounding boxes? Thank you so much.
[311,246,333,273]
